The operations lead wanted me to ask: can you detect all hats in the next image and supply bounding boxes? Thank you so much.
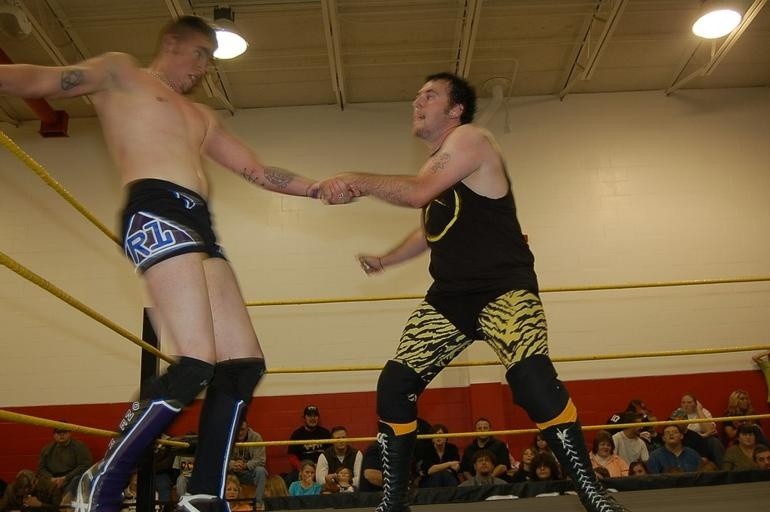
[304,404,319,417]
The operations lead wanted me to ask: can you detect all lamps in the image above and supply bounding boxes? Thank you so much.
[693,2,741,41]
[211,5,249,65]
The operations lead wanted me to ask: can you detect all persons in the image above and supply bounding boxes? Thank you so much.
[0,16,361,512]
[0,420,198,512]
[317,72,632,512]
[589,389,770,479]
[408,417,572,489]
[225,405,383,512]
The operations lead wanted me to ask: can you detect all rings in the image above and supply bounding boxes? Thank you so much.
[337,193,343,198]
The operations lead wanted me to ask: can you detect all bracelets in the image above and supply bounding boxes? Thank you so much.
[379,257,384,270]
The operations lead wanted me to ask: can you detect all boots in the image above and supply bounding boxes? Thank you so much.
[170,393,249,512]
[373,419,419,512]
[539,415,632,512]
[73,388,183,512]
